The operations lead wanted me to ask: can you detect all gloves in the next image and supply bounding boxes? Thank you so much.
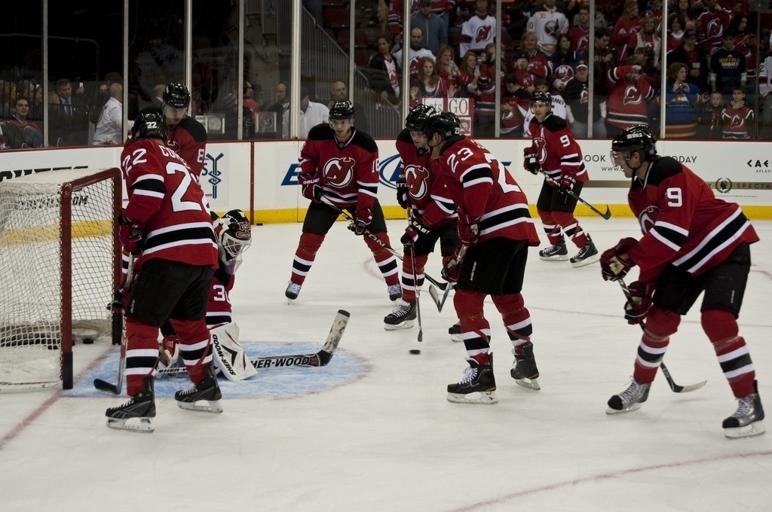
[401,220,432,246]
[397,178,411,210]
[117,214,142,248]
[348,212,373,237]
[601,236,638,281]
[558,174,577,194]
[299,173,322,203]
[624,281,657,326]
[455,206,482,248]
[442,257,465,285]
[523,147,541,176]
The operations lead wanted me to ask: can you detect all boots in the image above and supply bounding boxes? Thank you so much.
[445,354,497,392]
[388,282,401,300]
[721,380,766,428]
[173,377,222,404]
[509,341,541,379]
[607,380,651,411]
[384,301,417,323]
[539,240,569,257]
[284,281,302,300]
[104,392,158,419]
[570,241,599,263]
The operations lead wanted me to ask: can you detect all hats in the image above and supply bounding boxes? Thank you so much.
[575,56,590,69]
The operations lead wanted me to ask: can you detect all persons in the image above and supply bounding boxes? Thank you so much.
[599,125,764,428]
[105,106,223,419]
[524,91,599,263]
[285,99,402,301]
[159,209,252,382]
[115,81,207,285]
[0,0,772,150]
[421,110,541,394]
[383,104,458,325]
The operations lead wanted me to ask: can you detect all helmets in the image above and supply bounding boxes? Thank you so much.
[406,104,438,130]
[329,101,355,119]
[163,82,190,109]
[611,126,658,153]
[530,91,552,104]
[131,113,167,139]
[219,208,251,259]
[425,112,461,136]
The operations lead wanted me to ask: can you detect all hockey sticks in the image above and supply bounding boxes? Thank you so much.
[407,207,422,341]
[157,308,350,375]
[429,245,467,312]
[611,264,707,393]
[540,170,611,220]
[94,251,134,394]
[319,196,455,290]
